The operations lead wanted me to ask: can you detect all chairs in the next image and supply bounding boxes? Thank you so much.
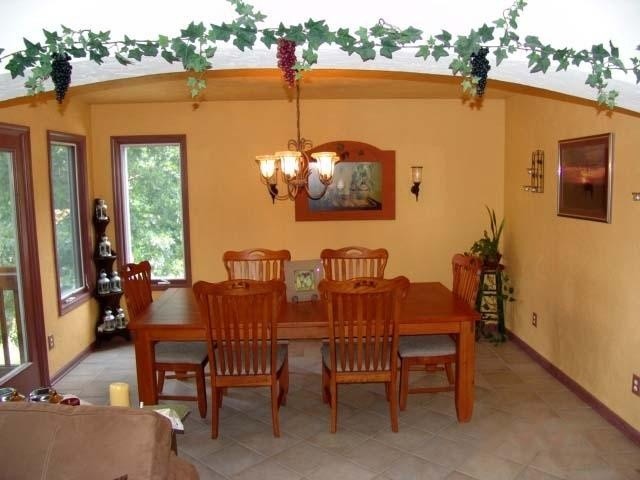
[117,261,223,419]
[397,254,480,411]
[193,280,289,439]
[319,276,411,433]
[223,249,292,281]
[319,246,388,281]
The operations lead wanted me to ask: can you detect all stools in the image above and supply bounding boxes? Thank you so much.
[474,266,508,343]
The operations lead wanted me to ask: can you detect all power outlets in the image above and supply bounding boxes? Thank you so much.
[532,312,537,328]
[631,374,640,396]
[48,335,54,351]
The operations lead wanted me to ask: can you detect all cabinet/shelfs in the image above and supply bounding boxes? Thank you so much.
[91,197,133,346]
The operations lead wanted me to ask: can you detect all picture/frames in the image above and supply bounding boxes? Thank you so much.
[283,258,323,303]
[294,140,397,221]
[556,131,612,224]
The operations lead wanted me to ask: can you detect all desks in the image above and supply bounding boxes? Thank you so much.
[128,282,483,422]
[20,391,192,456]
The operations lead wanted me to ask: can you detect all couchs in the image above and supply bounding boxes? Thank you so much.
[0,401,199,480]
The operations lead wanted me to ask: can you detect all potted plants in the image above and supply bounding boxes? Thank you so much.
[463,203,516,346]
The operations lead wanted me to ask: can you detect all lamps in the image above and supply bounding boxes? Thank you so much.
[410,166,423,203]
[256,67,340,200]
[267,168,278,205]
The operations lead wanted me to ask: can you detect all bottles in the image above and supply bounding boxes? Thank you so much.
[97,272,111,295]
[103,310,115,332]
[0,387,81,407]
[99,236,111,258]
[96,198,108,221]
[108,270,121,293]
[115,309,127,330]
